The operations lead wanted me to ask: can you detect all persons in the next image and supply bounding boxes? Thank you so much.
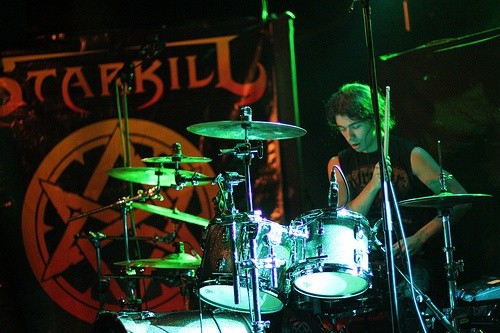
[326,83,468,333]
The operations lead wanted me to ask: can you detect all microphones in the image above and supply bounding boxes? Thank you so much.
[328,170,339,207]
[372,217,384,234]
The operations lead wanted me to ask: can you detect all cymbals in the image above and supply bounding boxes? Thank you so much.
[396,192,493,208]
[140,153,214,165]
[186,119,308,142]
[105,166,214,187]
[112,252,202,270]
[130,200,212,226]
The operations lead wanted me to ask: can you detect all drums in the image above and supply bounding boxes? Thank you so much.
[192,214,290,314]
[453,281,500,333]
[295,228,384,319]
[286,207,374,300]
[95,306,255,333]
